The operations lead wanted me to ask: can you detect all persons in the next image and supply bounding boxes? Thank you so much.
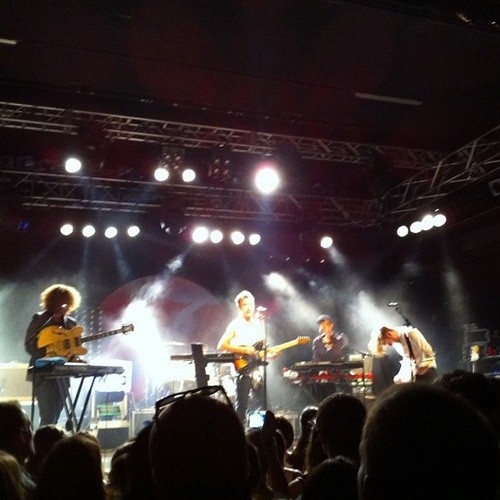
[218,290,281,425]
[24,284,87,429]
[374,326,437,381]
[1,372,500,500]
[311,314,353,398]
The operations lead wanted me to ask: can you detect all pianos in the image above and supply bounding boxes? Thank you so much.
[290,359,364,416]
[26,361,125,436]
[170,342,235,395]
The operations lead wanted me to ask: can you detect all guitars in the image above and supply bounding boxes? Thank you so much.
[36,320,134,362]
[233,335,311,376]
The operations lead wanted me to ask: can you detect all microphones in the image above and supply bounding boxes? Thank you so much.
[387,301,398,306]
[53,303,67,317]
[256,306,268,311]
